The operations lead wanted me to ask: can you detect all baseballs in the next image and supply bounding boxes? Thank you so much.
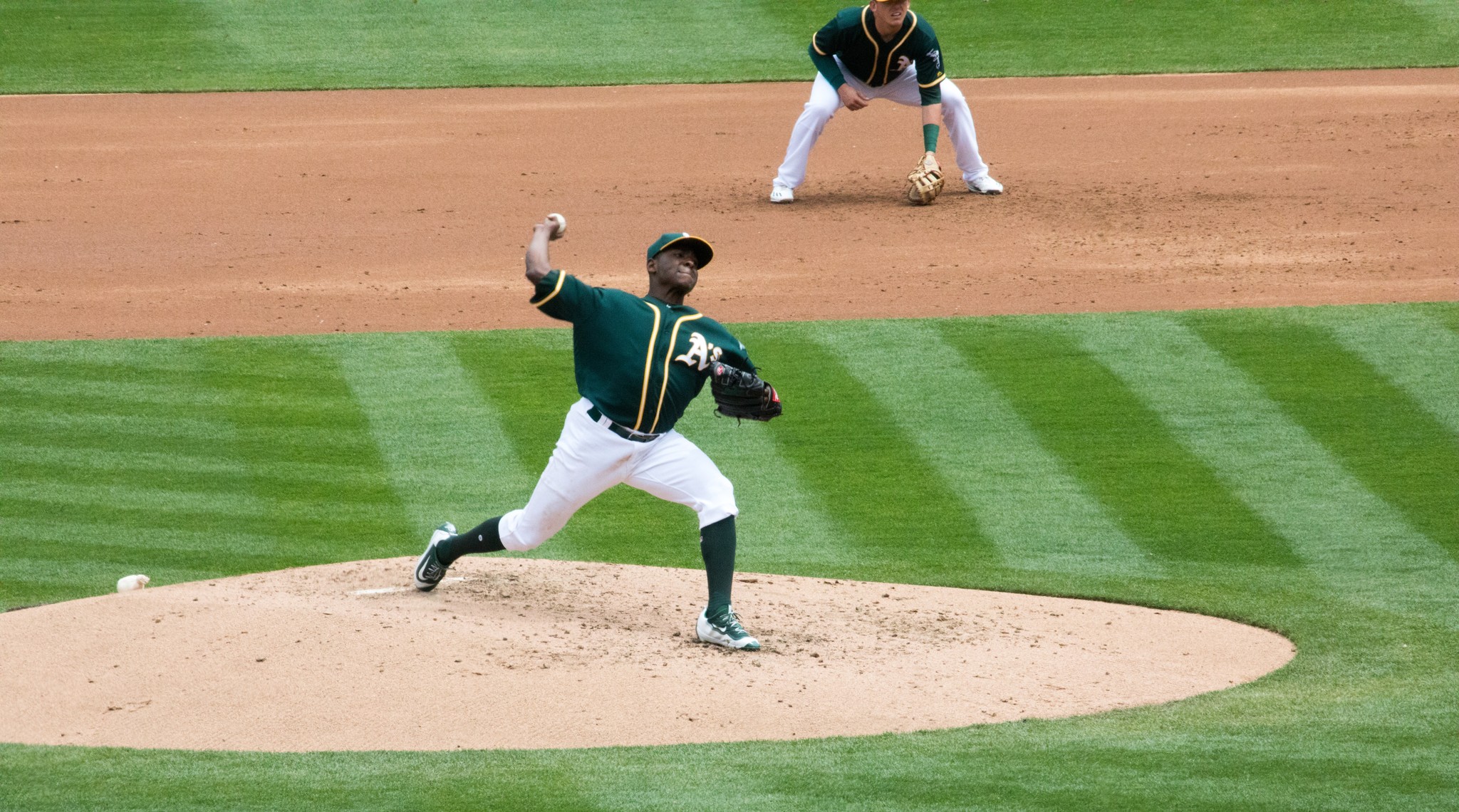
[544,213,567,234]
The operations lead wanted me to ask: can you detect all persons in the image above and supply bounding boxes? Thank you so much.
[413,214,782,651]
[770,0,1003,203]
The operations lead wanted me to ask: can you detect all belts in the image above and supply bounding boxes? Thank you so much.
[587,406,659,443]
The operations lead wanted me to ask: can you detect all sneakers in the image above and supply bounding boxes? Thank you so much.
[413,522,458,592]
[695,605,760,651]
[770,185,794,203]
[967,175,1003,196]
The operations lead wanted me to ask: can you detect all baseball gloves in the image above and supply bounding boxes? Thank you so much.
[709,362,783,422]
[907,154,945,204]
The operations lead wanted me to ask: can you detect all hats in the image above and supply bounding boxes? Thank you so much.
[648,232,714,270]
